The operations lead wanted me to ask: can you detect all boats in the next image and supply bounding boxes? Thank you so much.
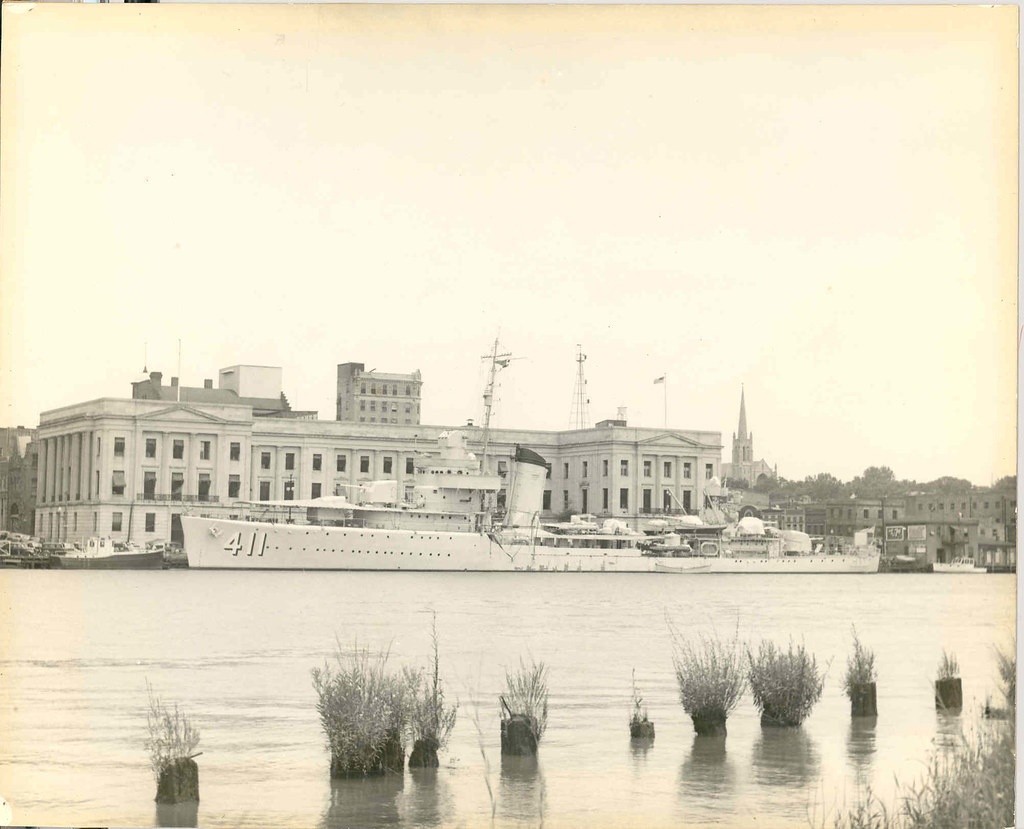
[972,537,1017,574]
[50,536,164,569]
[176,339,882,576]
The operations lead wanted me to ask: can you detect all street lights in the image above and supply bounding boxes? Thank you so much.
[286,473,296,524]
